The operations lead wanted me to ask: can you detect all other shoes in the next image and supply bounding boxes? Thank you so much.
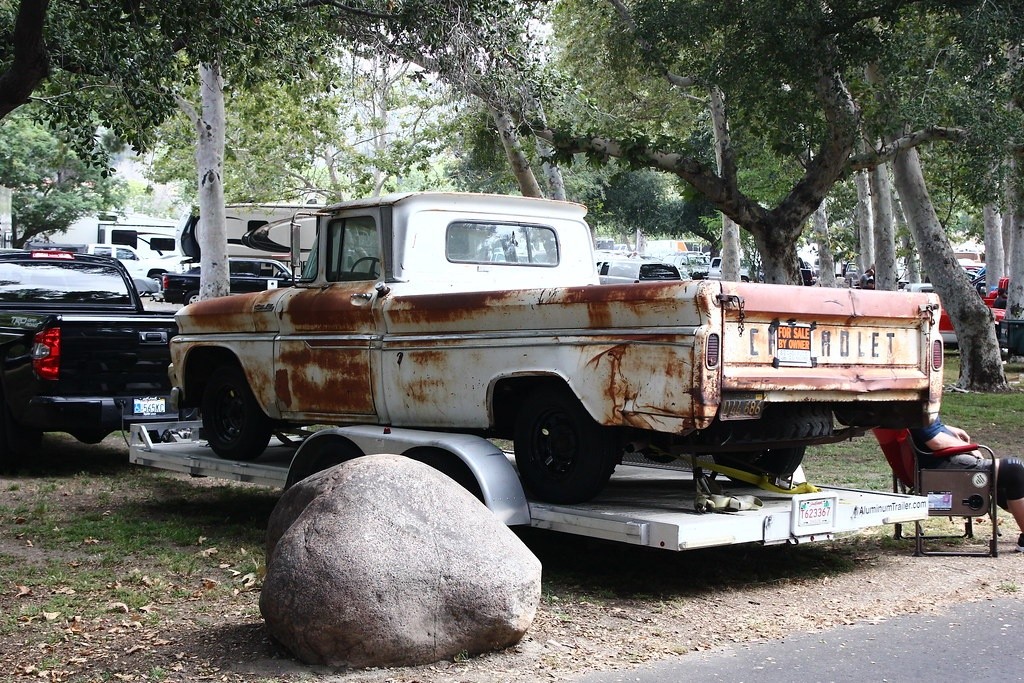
[1016,534,1024,552]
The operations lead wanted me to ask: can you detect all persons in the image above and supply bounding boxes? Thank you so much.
[911,413,1024,552]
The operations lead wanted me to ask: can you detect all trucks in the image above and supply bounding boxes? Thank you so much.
[25,223,184,291]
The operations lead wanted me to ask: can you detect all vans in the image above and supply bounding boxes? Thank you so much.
[662,251,711,279]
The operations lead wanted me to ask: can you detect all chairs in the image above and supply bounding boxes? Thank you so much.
[871,428,1002,557]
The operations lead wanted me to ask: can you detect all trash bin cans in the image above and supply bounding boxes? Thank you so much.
[997,318,1024,362]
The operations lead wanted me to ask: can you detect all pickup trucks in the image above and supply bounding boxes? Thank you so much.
[23,242,178,297]
[165,191,945,506]
[0,251,207,465]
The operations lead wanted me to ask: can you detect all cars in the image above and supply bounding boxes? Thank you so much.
[161,257,302,306]
[596,260,683,284]
[707,257,752,283]
